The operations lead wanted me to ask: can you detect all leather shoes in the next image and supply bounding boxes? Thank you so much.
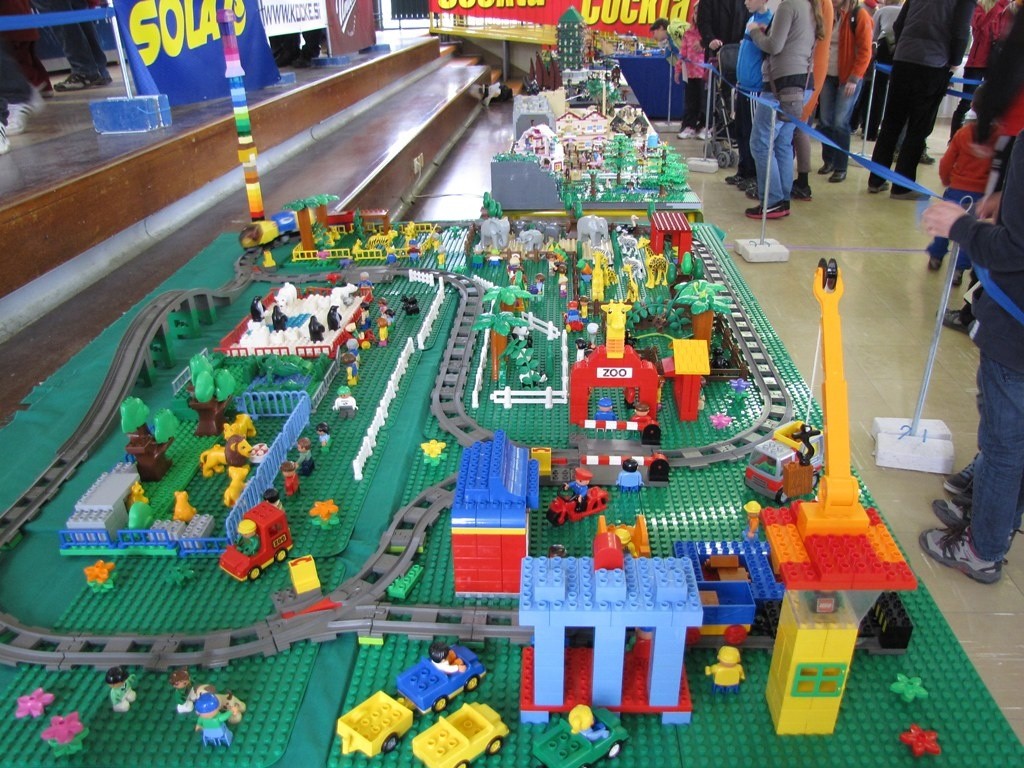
[829,169,847,181]
[818,162,836,174]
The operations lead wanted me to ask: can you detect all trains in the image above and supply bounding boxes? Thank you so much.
[236,206,357,254]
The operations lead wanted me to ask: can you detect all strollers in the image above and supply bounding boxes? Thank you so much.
[701,44,741,169]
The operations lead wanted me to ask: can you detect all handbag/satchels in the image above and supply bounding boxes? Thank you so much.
[776,86,803,121]
[988,40,1005,68]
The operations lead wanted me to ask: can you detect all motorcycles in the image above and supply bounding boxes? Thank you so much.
[546,484,609,527]
[562,312,584,333]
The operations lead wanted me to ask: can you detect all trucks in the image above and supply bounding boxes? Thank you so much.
[743,421,825,506]
[410,700,510,768]
[395,644,487,715]
[219,502,294,583]
[530,706,630,768]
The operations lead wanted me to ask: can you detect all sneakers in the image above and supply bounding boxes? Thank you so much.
[745,201,786,219]
[791,180,812,201]
[784,201,791,215]
[735,178,757,189]
[696,128,712,140]
[920,525,1000,583]
[932,496,969,531]
[95,70,111,84]
[0,124,10,154]
[935,307,969,335]
[4,103,33,134]
[53,70,102,90]
[945,454,980,494]
[677,127,696,139]
[747,187,761,199]
[920,152,935,164]
[725,175,744,184]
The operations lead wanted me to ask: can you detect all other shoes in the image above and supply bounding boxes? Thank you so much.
[868,182,889,192]
[891,189,928,200]
[951,269,964,287]
[928,256,942,270]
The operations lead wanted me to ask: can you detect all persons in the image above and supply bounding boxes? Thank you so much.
[234,519,261,556]
[566,296,591,333]
[432,226,443,252]
[534,252,567,297]
[705,646,746,695]
[574,339,588,360]
[359,297,396,348]
[919,0,1024,584]
[429,642,466,674]
[595,397,652,422]
[279,437,314,495]
[106,666,137,712]
[264,488,284,510]
[342,339,360,387]
[649,0,977,219]
[407,240,420,265]
[269,28,327,68]
[564,458,645,510]
[472,245,504,268]
[357,272,374,295]
[568,704,609,741]
[332,386,359,420]
[168,664,239,748]
[316,421,330,454]
[0,0,115,157]
